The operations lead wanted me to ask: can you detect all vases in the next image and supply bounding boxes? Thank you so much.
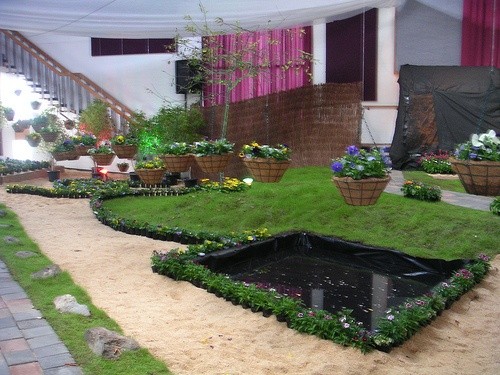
[242,159,290,183]
[73,146,96,156]
[134,171,165,186]
[191,154,229,178]
[453,161,500,197]
[50,153,78,161]
[111,144,137,160]
[332,177,392,207]
[160,156,192,172]
[91,153,115,166]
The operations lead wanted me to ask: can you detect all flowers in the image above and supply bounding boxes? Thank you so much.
[5,128,500,355]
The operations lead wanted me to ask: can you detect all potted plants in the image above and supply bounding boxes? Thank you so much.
[12,119,30,140]
[64,120,74,130]
[31,101,41,110]
[30,116,49,134]
[3,107,14,121]
[0,156,51,176]
[27,133,41,146]
[39,129,70,180]
[39,128,59,142]
